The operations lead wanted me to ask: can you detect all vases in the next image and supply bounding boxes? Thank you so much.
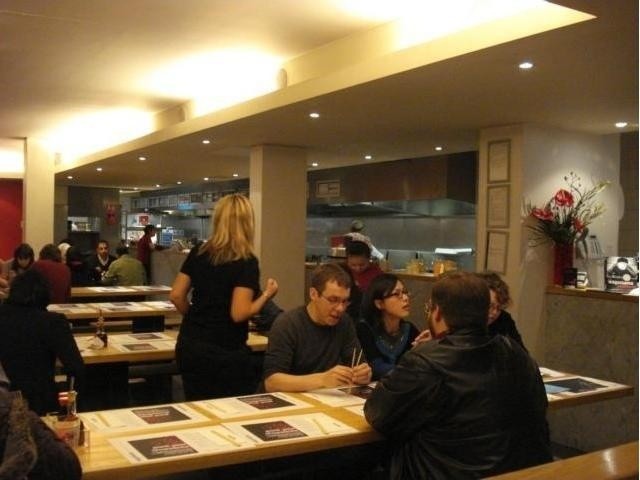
[552,236,575,289]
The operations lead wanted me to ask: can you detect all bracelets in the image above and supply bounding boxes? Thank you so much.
[263,290,272,301]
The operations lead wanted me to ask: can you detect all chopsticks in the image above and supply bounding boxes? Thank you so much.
[348,348,363,393]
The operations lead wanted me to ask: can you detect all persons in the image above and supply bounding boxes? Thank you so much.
[83,240,117,279]
[0,272,93,417]
[115,239,128,256]
[139,224,158,285]
[7,243,34,280]
[343,243,383,302]
[1,258,12,291]
[257,265,372,392]
[30,243,73,304]
[57,238,80,267]
[477,274,529,352]
[357,274,422,381]
[98,246,148,302]
[364,270,553,480]
[342,220,384,260]
[169,193,279,400]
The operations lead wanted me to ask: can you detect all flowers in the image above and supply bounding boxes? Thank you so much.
[521,168,611,248]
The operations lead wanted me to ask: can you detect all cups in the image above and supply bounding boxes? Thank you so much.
[46,411,60,428]
[73,429,91,472]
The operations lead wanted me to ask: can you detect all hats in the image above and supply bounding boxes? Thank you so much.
[142,224,158,232]
[351,221,366,231]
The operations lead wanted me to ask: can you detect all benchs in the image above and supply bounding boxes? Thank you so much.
[469,436,640,480]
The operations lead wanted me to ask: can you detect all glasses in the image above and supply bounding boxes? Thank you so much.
[382,287,409,298]
[315,288,353,306]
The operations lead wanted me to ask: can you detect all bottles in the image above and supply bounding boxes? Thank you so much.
[95,314,107,348]
[68,388,85,447]
[56,391,67,440]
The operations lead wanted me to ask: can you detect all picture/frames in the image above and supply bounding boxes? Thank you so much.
[605,254,638,288]
